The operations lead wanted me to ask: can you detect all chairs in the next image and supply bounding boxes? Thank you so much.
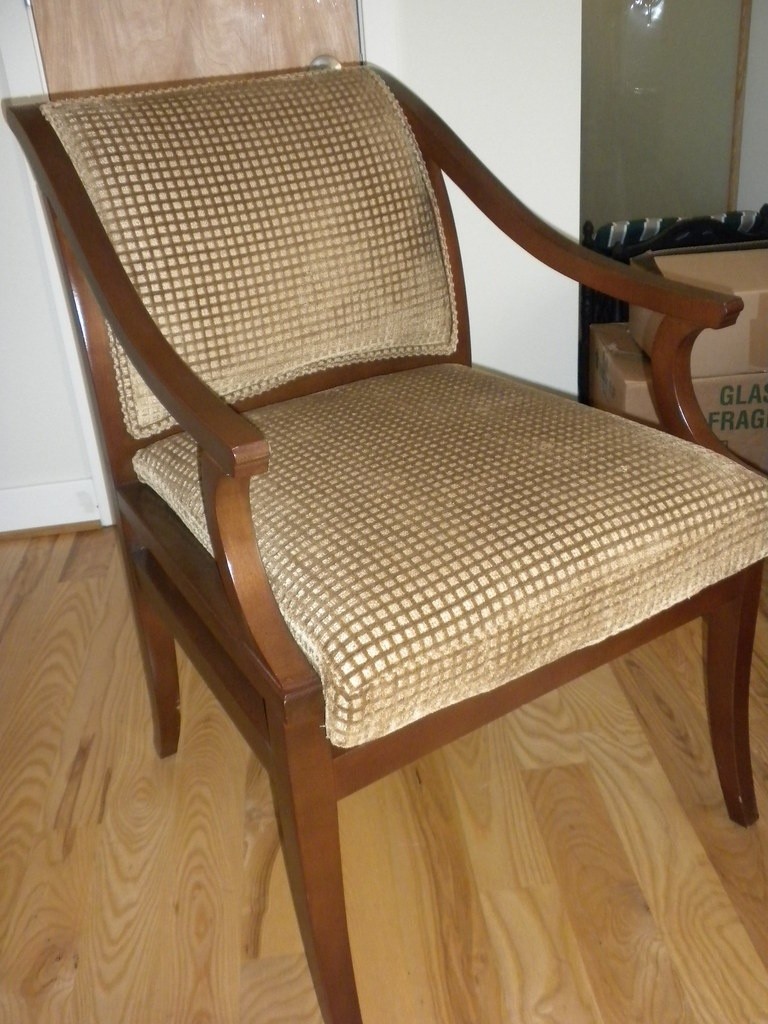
[1,53,767,1018]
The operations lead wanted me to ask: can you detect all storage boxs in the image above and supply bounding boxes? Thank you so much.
[587,235,768,473]
